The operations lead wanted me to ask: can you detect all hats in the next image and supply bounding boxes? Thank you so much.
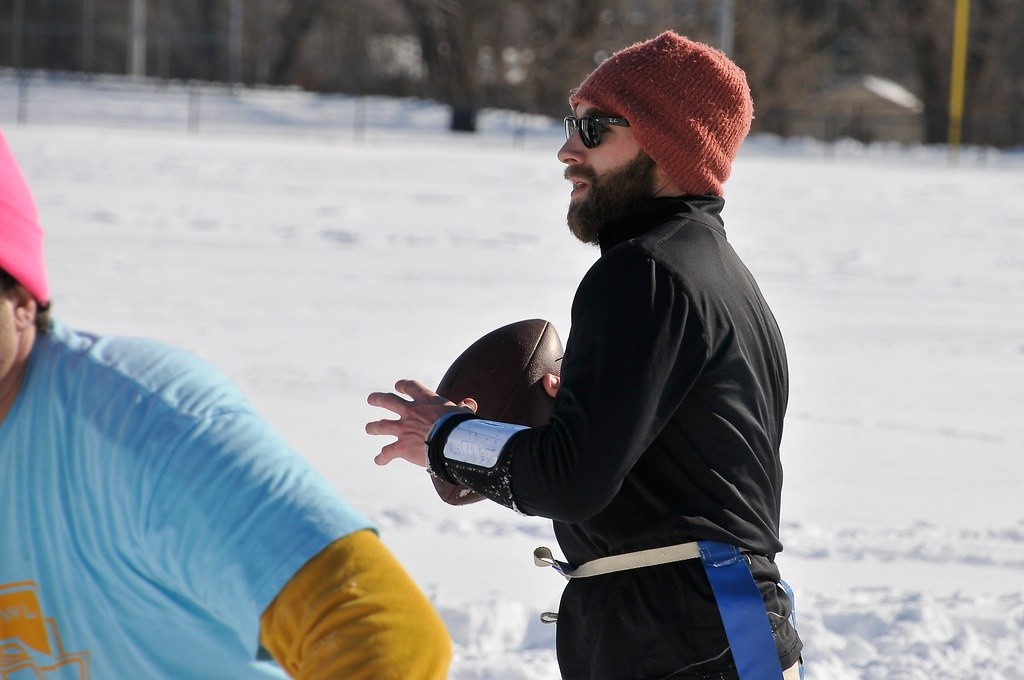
[1,133,48,308]
[568,28,754,196]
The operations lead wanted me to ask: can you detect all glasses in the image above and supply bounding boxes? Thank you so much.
[564,115,629,149]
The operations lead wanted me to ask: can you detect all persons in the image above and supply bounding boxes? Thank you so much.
[400,0,482,134]
[0,120,453,680]
[364,31,804,680]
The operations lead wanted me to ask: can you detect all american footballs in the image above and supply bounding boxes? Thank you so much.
[427,318,565,506]
